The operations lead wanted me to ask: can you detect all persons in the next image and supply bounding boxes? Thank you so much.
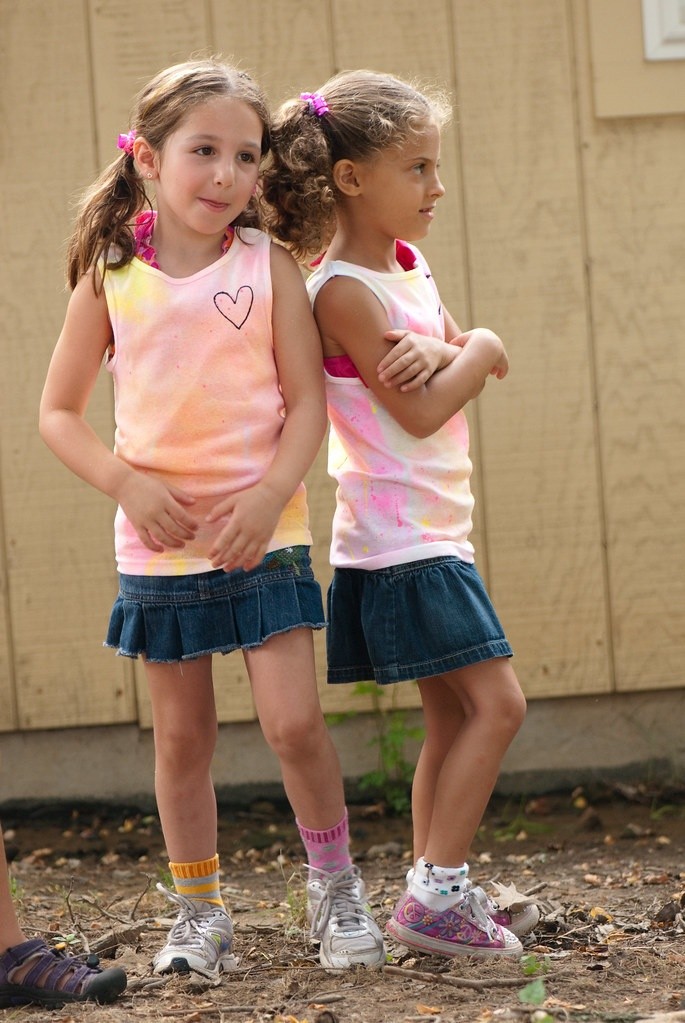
[257,68,540,961]
[37,60,388,979]
[0,825,128,1009]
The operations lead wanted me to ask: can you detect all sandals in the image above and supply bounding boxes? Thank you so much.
[0,939,127,1009]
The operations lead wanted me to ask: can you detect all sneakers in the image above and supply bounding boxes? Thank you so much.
[152,883,235,989]
[302,863,387,976]
[386,889,523,963]
[459,879,540,940]
